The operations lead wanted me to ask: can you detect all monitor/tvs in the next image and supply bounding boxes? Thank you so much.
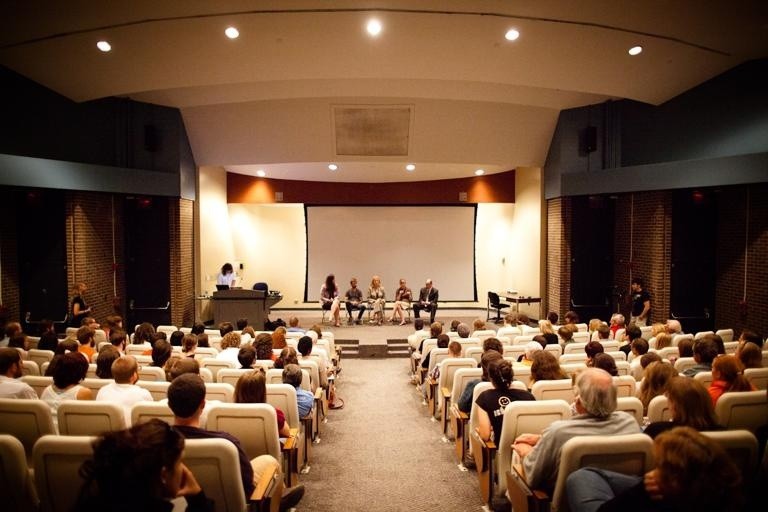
[216,285,229,290]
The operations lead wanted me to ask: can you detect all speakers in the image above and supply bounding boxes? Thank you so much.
[145,126,158,152]
[582,127,597,152]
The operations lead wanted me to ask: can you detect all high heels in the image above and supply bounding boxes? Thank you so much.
[328,317,333,322]
[387,317,395,323]
[377,320,382,326]
[399,319,406,326]
[334,322,340,327]
[370,315,376,322]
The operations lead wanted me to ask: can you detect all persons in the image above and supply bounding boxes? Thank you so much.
[387,279,413,325]
[531,351,570,380]
[95,357,152,429]
[475,362,537,449]
[0,348,39,399]
[546,312,559,324]
[241,326,255,343]
[238,346,258,369]
[456,323,470,337]
[670,338,694,364]
[457,351,504,414]
[652,320,681,350]
[708,354,759,409]
[559,327,578,352]
[585,342,605,359]
[282,364,314,420]
[408,319,430,349]
[219,322,232,336]
[298,337,330,387]
[413,279,439,324]
[9,334,29,350]
[156,356,227,429]
[181,335,198,355]
[643,375,721,437]
[149,338,173,367]
[171,331,183,346]
[167,374,305,512]
[532,336,548,347]
[236,318,248,330]
[610,313,625,335]
[481,338,504,353]
[213,332,241,366]
[133,323,154,344]
[639,362,680,411]
[640,352,663,369]
[511,368,640,487]
[585,352,618,375]
[629,278,650,327]
[345,277,366,324]
[310,325,322,338]
[420,334,450,367]
[565,426,748,512]
[218,263,236,289]
[320,275,342,326]
[151,330,166,347]
[619,327,643,356]
[496,314,521,341]
[38,317,108,354]
[198,334,208,347]
[629,338,649,362]
[234,371,290,436]
[75,419,214,512]
[288,317,304,333]
[518,341,543,361]
[253,333,277,361]
[110,332,126,350]
[739,330,763,349]
[368,277,385,325]
[191,321,205,334]
[275,326,285,333]
[96,349,121,378]
[272,330,287,348]
[42,352,92,400]
[516,313,535,335]
[1,322,22,347]
[734,340,762,375]
[306,329,318,345]
[688,340,719,375]
[565,310,579,324]
[705,333,725,354]
[451,321,463,331]
[473,319,486,329]
[105,316,125,330]
[274,347,298,369]
[419,321,442,354]
[589,318,608,341]
[71,282,92,325]
[422,341,462,404]
[533,319,558,346]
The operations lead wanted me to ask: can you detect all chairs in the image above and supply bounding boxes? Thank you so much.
[203,328,221,338]
[486,291,510,323]
[564,343,586,353]
[498,328,522,336]
[700,431,760,468]
[368,304,385,323]
[205,404,299,488]
[268,369,323,445]
[744,367,768,388]
[513,366,531,390]
[320,332,343,372]
[673,356,697,370]
[286,332,305,339]
[194,347,216,363]
[574,323,588,333]
[424,349,449,417]
[345,308,359,320]
[450,379,531,475]
[725,341,740,354]
[218,367,253,384]
[253,281,268,296]
[317,339,340,381]
[266,385,308,471]
[66,327,81,340]
[591,329,614,342]
[418,338,441,394]
[559,352,592,365]
[448,337,479,346]
[136,382,171,400]
[715,390,768,427]
[126,343,151,356]
[531,381,572,405]
[615,328,628,340]
[33,434,101,510]
[180,327,192,336]
[20,360,39,376]
[524,327,542,335]
[27,348,55,365]
[514,335,535,345]
[658,346,681,361]
[204,381,236,403]
[644,394,677,426]
[695,370,714,385]
[612,375,639,399]
[256,359,274,370]
[397,303,411,323]
[616,397,644,426]
[716,329,734,340]
[79,378,116,394]
[447,330,459,342]
[572,331,591,344]
[156,325,178,340]
[19,375,57,395]
[632,362,672,382]
[200,358,233,369]
[134,356,152,366]
[1,399,55,463]
[322,308,335,324]
[472,399,573,504]
[560,365,586,386]
[472,330,497,341]
[129,333,136,343]
[94,329,107,349]
[461,346,484,365]
[440,359,478,436]
[139,366,166,382]
[613,360,632,376]
[271,348,284,357]
[201,368,212,381]
[503,346,525,361]
[129,404,204,428]
[505,433,657,511]
[607,350,627,360]
[176,437,283,511]
[0,435,40,511]
[27,336,41,350]
[254,330,274,336]
[545,343,563,358]
[600,339,619,352]
[499,335,513,346]
[301,362,328,421]
[695,331,715,340]
[672,333,693,347]
[445,368,482,445]
[56,401,128,436]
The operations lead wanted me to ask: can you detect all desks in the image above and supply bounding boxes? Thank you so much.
[506,294,541,325]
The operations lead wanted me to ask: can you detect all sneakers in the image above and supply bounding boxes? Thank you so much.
[347,318,354,326]
[356,319,364,325]
